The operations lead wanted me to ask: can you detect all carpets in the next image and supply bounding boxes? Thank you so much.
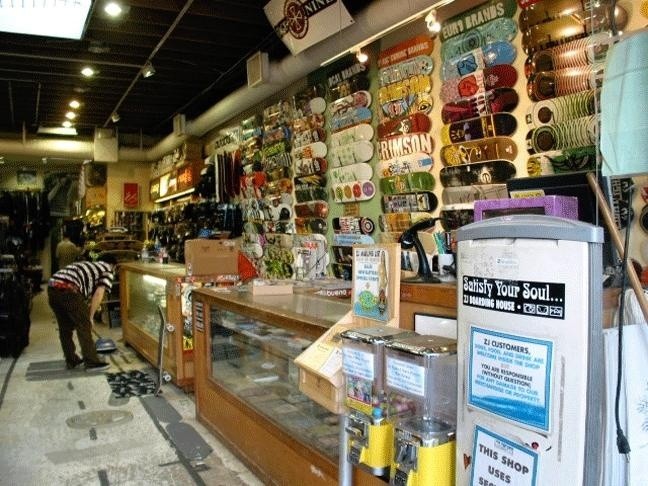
[104,369,163,400]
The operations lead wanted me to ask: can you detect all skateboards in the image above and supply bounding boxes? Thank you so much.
[239,0,636,287]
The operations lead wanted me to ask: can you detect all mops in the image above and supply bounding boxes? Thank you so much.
[91,327,118,354]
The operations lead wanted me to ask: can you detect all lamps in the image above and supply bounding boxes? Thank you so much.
[142,61,156,79]
[111,112,120,123]
[424,10,441,34]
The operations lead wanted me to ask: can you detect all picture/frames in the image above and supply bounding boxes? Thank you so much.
[122,181,140,209]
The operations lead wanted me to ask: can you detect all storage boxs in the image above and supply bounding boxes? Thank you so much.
[184,239,240,275]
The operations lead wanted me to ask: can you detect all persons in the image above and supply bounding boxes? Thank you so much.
[46,253,118,373]
[54,230,82,269]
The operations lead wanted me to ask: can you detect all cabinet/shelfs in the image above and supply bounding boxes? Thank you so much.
[120,262,458,486]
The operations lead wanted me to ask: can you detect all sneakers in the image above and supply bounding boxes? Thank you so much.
[86,362,112,373]
[67,359,84,369]
